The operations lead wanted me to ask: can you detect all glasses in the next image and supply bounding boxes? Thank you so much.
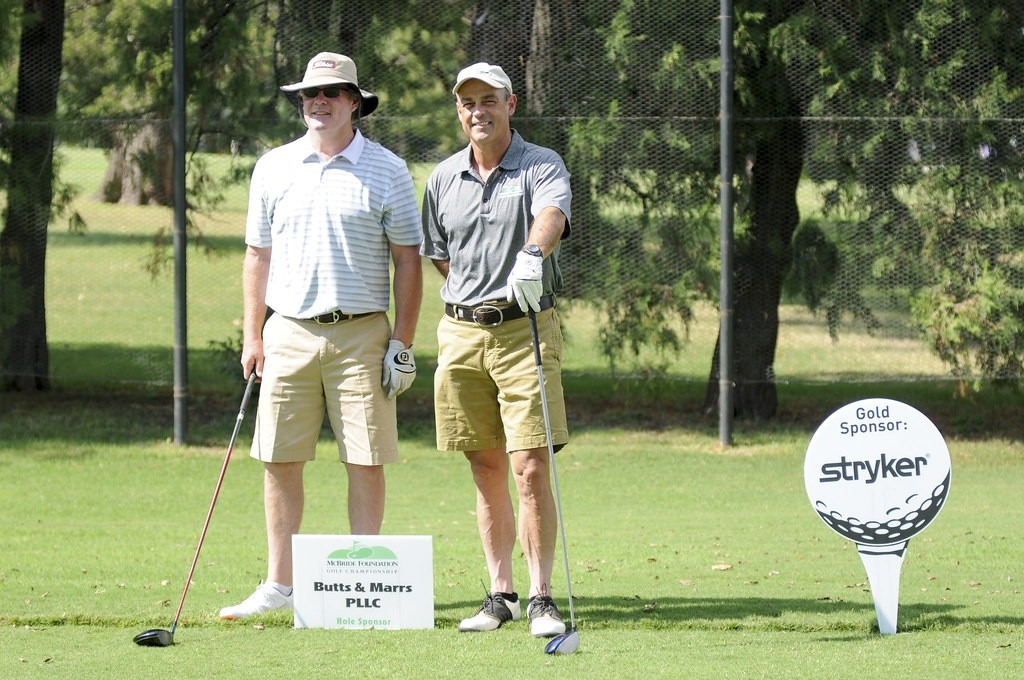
[300,85,349,99]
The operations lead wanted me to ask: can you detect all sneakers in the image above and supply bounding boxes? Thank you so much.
[458,578,521,632]
[218,579,293,621]
[526,583,566,637]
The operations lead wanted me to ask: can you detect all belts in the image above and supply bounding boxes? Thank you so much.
[304,309,380,325]
[445,295,555,328]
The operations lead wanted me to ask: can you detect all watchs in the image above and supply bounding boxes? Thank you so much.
[522,244,543,258]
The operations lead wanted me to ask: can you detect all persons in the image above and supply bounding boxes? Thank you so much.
[219,52,423,621]
[420,62,573,638]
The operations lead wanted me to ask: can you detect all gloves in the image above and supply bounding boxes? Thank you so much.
[506,251,544,313]
[381,339,417,402]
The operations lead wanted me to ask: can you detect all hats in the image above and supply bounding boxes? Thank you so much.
[280,51,379,120]
[451,61,514,96]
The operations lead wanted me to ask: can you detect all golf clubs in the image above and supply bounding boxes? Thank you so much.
[528,301,580,655]
[133,369,257,647]
